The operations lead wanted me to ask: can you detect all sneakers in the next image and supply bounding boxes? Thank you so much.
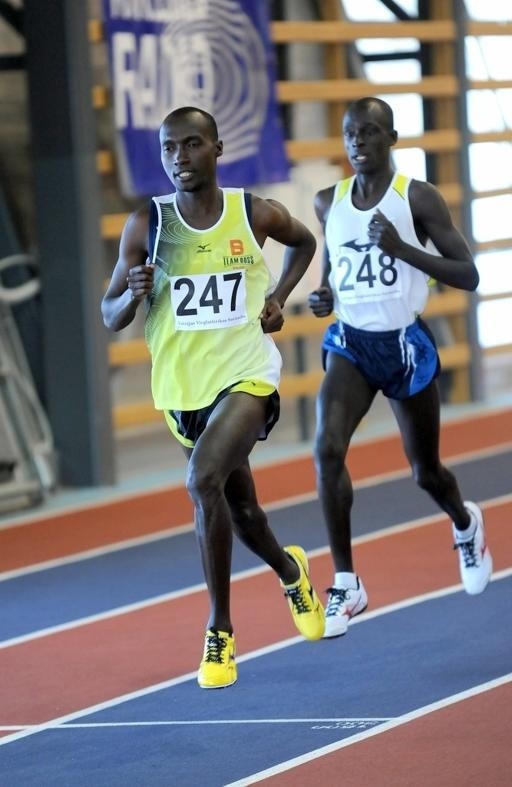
[453,500,493,595]
[324,574,368,639]
[280,545,325,641]
[197,629,237,689]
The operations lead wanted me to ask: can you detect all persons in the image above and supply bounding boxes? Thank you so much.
[307,96,492,637]
[100,107,327,691]
